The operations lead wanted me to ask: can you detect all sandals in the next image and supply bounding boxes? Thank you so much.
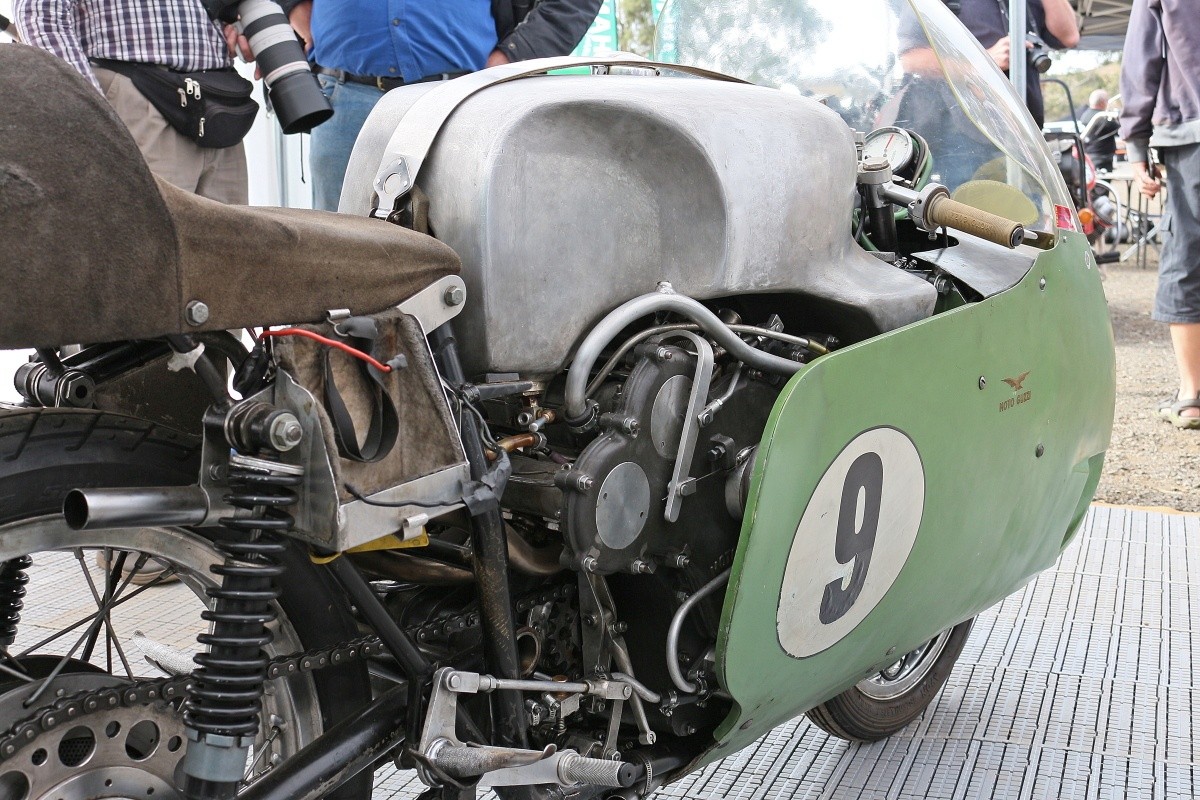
[1156,398,1200,427]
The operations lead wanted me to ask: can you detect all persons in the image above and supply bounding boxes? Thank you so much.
[10,0,260,584]
[896,0,1079,196]
[1080,90,1120,197]
[1117,0,1200,428]
[274,0,604,212]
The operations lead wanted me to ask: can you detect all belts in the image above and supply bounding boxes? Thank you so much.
[311,65,473,91]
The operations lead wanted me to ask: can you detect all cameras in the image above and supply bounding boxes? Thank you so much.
[1025,33,1052,73]
[199,0,335,136]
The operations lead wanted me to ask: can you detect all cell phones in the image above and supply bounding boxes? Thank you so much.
[1147,148,1156,199]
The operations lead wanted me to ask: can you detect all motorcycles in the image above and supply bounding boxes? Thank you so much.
[0,0,1117,800]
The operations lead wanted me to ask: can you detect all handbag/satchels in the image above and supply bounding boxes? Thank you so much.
[88,57,259,148]
